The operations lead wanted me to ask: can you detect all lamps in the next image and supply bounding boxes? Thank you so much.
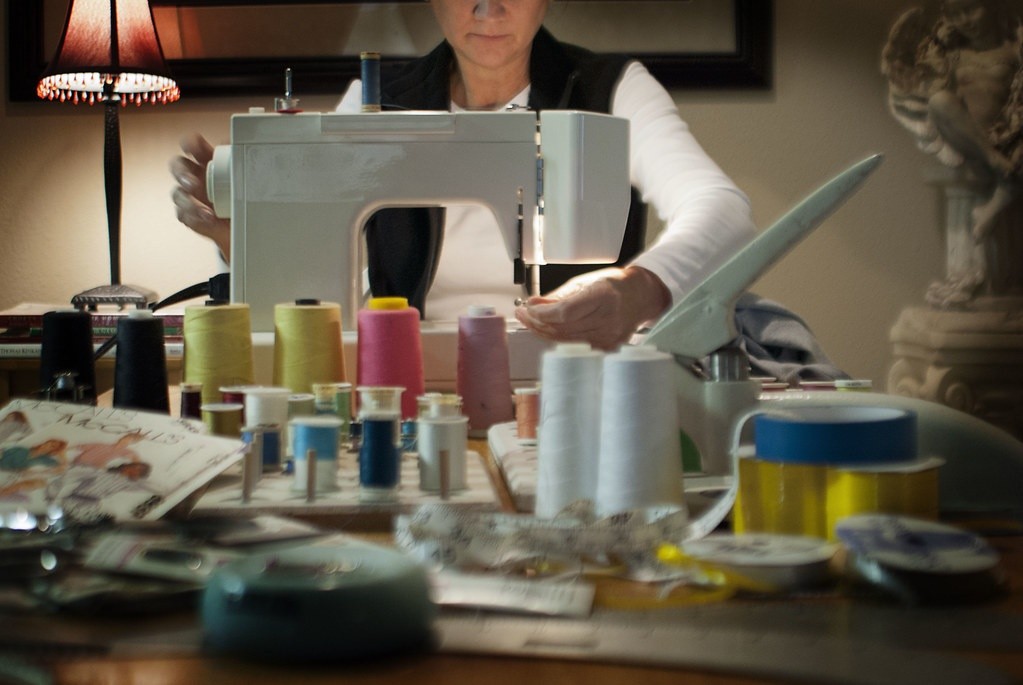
[38,0,181,313]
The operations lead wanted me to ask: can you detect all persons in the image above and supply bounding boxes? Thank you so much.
[930,0,1023,242]
[170,1,754,354]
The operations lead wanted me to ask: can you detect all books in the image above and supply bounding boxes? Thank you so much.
[0,302,184,357]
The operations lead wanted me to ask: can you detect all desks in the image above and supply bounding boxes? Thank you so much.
[0,389,1023,685]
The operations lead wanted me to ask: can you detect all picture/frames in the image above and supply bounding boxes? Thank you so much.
[6,0,774,106]
